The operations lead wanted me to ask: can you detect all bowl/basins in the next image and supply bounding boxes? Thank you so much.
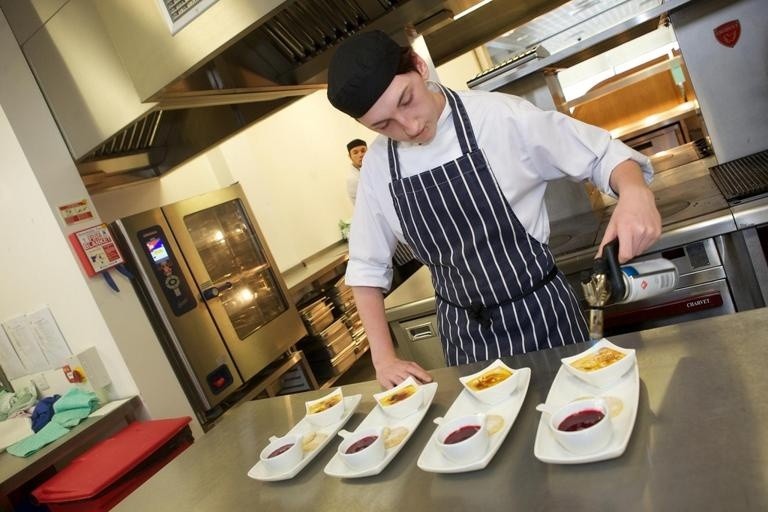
[560,336,636,387]
[373,376,426,420]
[306,384,343,424]
[439,413,486,459]
[259,435,303,473]
[339,427,384,467]
[461,358,519,403]
[549,398,613,448]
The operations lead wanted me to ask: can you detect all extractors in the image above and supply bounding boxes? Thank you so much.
[79,109,163,162]
[238,2,401,72]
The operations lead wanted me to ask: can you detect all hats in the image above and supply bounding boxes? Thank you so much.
[347,139,366,151]
[328,29,401,118]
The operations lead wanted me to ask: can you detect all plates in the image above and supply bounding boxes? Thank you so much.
[250,392,360,480]
[324,378,438,479]
[417,363,532,475]
[534,363,641,464]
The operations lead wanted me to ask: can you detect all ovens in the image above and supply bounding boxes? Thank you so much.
[565,237,739,339]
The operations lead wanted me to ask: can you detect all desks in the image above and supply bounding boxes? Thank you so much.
[0,395,141,511]
[109,308,766,512]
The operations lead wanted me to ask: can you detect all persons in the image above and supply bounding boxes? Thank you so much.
[345,138,421,280]
[323,28,663,392]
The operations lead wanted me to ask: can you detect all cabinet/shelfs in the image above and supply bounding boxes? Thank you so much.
[544,8,724,207]
